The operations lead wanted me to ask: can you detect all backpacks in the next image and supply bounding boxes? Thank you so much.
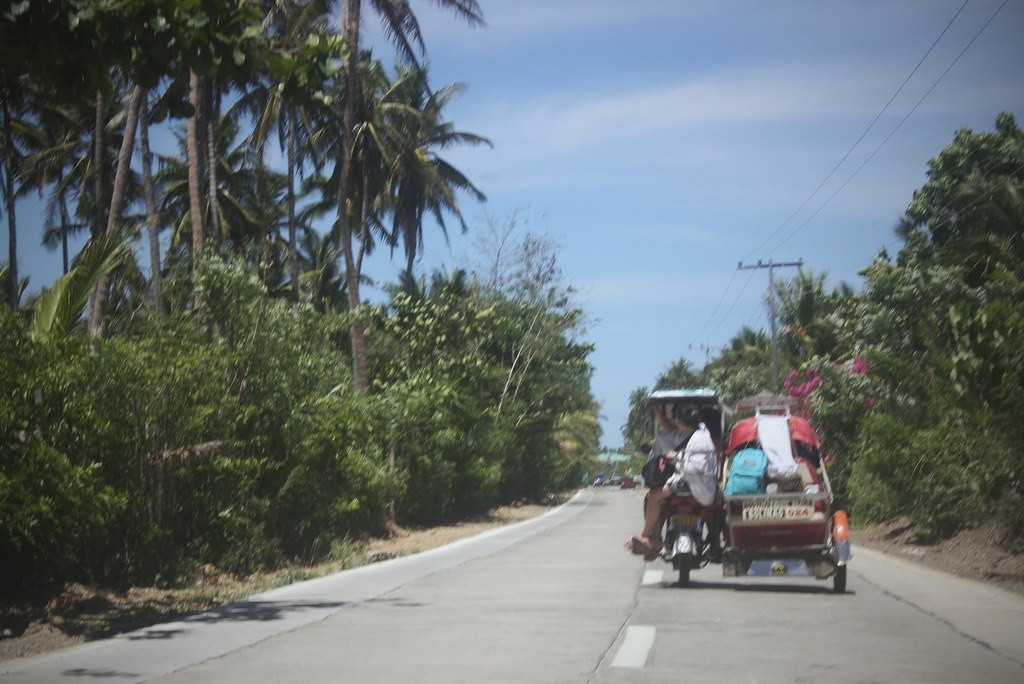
[722,448,768,497]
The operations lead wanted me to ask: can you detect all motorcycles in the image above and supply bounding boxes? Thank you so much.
[647,388,853,591]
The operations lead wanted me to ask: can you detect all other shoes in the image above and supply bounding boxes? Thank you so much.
[623,535,652,554]
[645,538,664,562]
[709,544,723,564]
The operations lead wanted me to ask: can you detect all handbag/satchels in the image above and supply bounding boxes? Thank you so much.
[642,453,676,489]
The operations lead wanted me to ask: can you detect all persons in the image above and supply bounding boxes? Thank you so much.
[626,401,719,561]
